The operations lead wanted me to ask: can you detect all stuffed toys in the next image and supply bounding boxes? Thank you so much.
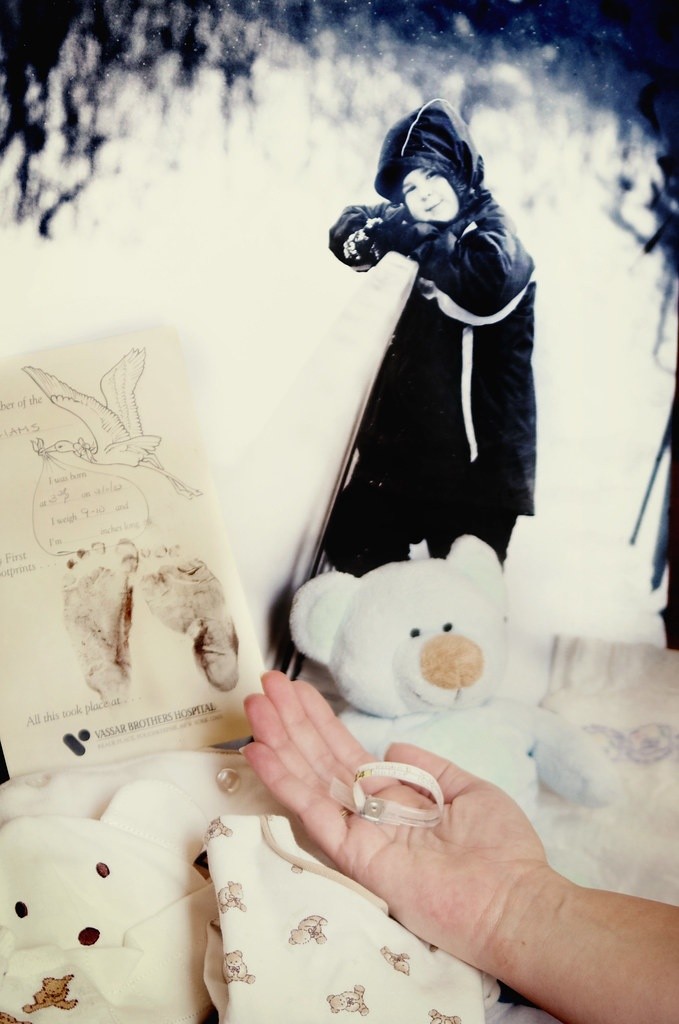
[288,534,539,820]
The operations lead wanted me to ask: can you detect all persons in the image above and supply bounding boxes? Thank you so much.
[228,669,679,1024]
[288,99,535,668]
